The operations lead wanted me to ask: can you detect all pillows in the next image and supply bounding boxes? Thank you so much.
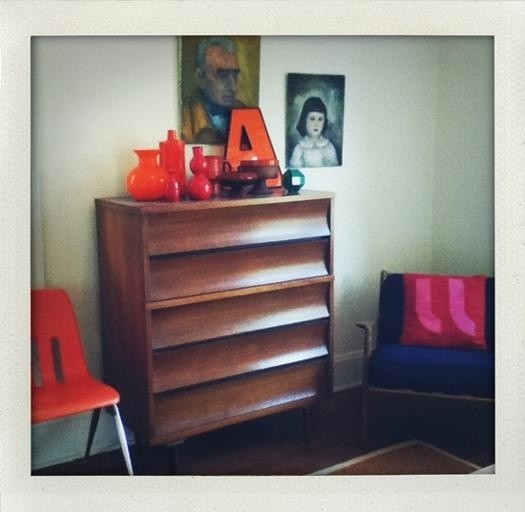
[399,272,486,349]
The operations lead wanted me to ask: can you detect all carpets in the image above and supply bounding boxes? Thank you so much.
[302,439,485,476]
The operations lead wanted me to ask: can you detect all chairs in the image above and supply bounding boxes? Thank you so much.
[357,271,495,465]
[31,288,135,475]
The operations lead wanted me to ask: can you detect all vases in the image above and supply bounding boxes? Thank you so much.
[127,129,221,203]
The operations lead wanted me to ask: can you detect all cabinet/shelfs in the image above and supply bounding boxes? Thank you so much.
[94,189,335,475]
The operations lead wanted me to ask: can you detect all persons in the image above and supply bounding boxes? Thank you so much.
[287,93,343,170]
[180,35,249,146]
